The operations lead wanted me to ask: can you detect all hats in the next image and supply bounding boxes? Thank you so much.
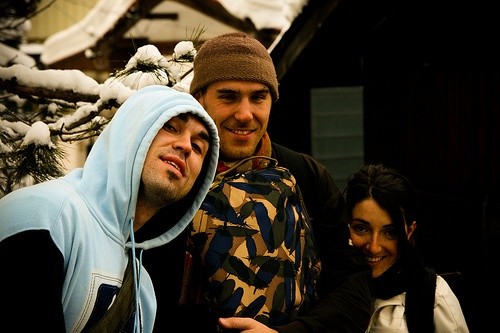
[188,31,279,103]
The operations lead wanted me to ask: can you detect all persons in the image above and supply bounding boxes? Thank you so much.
[0,86,221,331]
[343,164,469,333]
[145,32,372,333]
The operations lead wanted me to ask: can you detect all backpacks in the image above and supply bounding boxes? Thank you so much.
[182,155,321,328]
